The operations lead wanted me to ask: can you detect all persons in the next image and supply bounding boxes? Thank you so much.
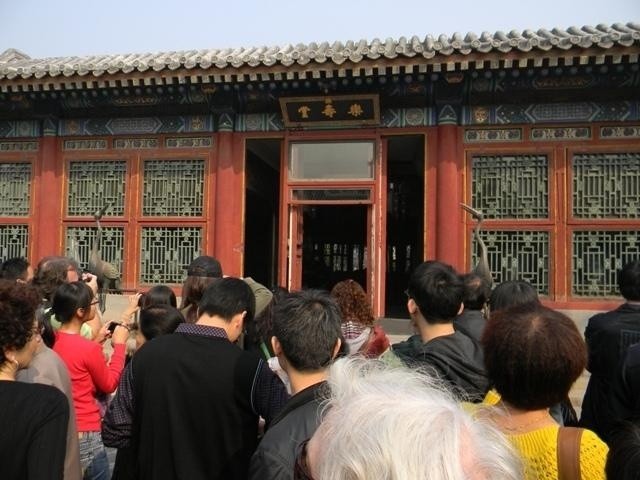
[250,290,341,480]
[180,254,272,326]
[488,280,577,426]
[28,257,105,341]
[395,259,489,401]
[0,256,35,284]
[330,280,389,360]
[452,270,488,340]
[14,322,81,479]
[303,358,520,480]
[38,280,130,479]
[450,303,610,480]
[100,276,253,479]
[136,305,179,352]
[0,298,69,480]
[582,262,639,476]
[101,286,177,362]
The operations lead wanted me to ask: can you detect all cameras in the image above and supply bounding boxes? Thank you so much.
[108,322,121,333]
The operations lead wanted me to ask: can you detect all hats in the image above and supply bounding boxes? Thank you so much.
[187,256,223,277]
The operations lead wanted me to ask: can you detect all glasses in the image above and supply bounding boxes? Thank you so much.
[404,288,411,299]
[86,299,102,309]
[30,323,48,335]
